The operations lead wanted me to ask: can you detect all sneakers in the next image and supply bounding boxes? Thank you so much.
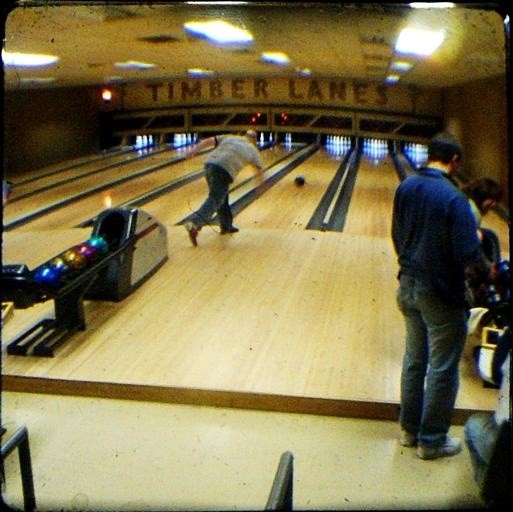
[185,223,197,246]
[400,431,461,459]
[221,227,238,234]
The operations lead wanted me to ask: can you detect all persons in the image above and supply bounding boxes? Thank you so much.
[391,131,483,459]
[181,129,264,247]
[461,177,504,313]
[464,324,512,491]
